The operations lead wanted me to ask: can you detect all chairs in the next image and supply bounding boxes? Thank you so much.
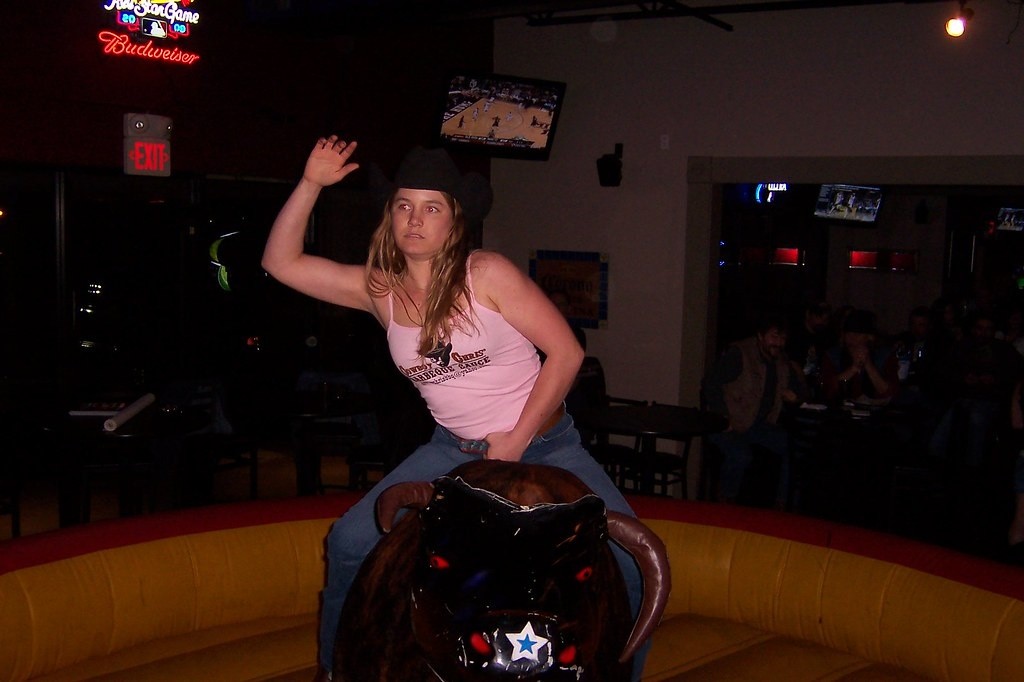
[566,357,621,485]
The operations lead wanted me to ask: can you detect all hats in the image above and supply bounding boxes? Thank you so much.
[392,145,460,198]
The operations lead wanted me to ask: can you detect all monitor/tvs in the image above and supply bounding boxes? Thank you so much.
[810,180,889,224]
[435,66,567,165]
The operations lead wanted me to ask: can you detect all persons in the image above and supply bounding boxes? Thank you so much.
[790,298,1023,544]
[260,135,654,682]
[454,78,557,139]
[702,310,793,465]
[827,190,860,218]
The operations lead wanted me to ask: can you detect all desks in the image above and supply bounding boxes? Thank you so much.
[581,402,730,497]
[781,402,925,524]
[36,401,224,521]
[243,399,397,498]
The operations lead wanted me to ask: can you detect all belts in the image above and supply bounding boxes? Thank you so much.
[451,403,564,454]
